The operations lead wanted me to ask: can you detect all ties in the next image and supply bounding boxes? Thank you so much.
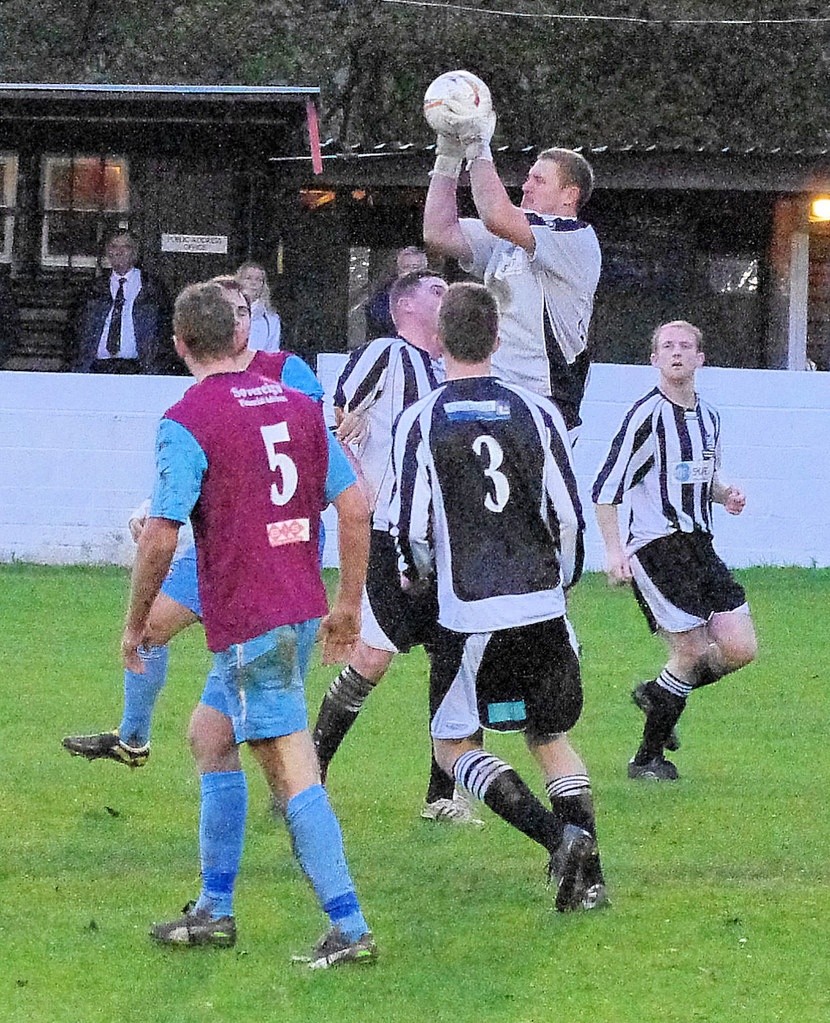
[105,277,127,356]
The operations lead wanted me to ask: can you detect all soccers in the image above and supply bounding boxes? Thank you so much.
[422,68,493,141]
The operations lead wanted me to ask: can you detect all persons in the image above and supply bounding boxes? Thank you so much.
[119,283,381,976]
[366,245,430,338]
[589,321,757,779]
[422,73,603,454]
[234,262,282,354]
[308,268,480,827]
[59,275,324,766]
[385,280,611,915]
[73,228,173,374]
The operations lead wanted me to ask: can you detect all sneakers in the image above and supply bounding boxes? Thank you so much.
[544,825,596,913]
[419,788,485,823]
[148,899,236,948]
[581,876,612,912]
[292,924,378,971]
[61,728,151,769]
[630,681,680,751]
[627,753,680,780]
